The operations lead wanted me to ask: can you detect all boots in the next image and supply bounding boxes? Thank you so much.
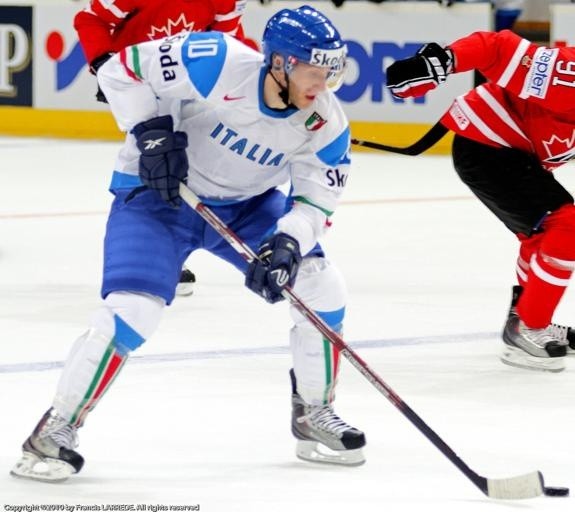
[23,406,83,472]
[503,286,575,357]
[289,368,365,449]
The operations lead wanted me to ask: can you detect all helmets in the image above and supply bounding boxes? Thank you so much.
[262,6,348,92]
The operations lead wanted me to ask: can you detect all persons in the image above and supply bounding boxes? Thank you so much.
[381,26,575,359]
[70,0,262,106]
[20,5,368,473]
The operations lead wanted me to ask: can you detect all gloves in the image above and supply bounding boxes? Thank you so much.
[387,43,454,98]
[129,115,189,207]
[245,233,302,303]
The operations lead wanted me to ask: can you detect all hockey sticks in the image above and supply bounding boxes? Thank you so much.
[349,124,450,157]
[177,182,545,498]
